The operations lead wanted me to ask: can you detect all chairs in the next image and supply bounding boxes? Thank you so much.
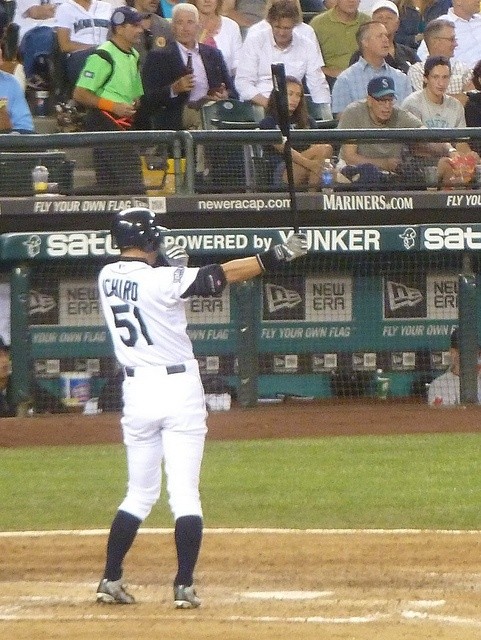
[200,99,266,194]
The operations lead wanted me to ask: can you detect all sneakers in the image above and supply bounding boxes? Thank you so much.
[174,584,201,609]
[97,576,138,604]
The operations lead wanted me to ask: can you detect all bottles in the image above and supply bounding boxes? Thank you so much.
[182,52,194,97]
[320,157,335,193]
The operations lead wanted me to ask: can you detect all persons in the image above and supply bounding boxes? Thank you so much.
[349,1,423,74]
[331,21,412,119]
[54,0,113,82]
[260,76,352,192]
[309,0,373,95]
[72,7,151,195]
[337,76,460,186]
[127,0,173,70]
[406,19,479,102]
[0,49,39,134]
[465,60,481,158]
[13,0,62,91]
[96,208,307,609]
[0,335,77,418]
[395,0,452,48]
[416,1,481,69]
[217,0,269,41]
[233,0,329,108]
[401,56,481,189]
[142,3,239,131]
[427,329,480,407]
[188,0,242,79]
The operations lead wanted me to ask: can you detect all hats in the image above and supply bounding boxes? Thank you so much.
[0,337,12,351]
[372,1,401,15]
[368,77,398,98]
[424,56,451,70]
[111,6,152,25]
[451,329,481,348]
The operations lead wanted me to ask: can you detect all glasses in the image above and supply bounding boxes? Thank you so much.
[435,37,458,41]
[373,97,397,104]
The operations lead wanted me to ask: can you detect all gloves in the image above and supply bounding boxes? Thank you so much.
[163,245,189,267]
[273,233,308,263]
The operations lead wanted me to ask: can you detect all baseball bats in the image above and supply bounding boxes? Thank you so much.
[270,64,298,235]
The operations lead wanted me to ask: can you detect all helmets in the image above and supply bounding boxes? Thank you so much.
[111,208,168,252]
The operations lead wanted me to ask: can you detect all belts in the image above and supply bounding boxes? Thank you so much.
[125,364,185,376]
